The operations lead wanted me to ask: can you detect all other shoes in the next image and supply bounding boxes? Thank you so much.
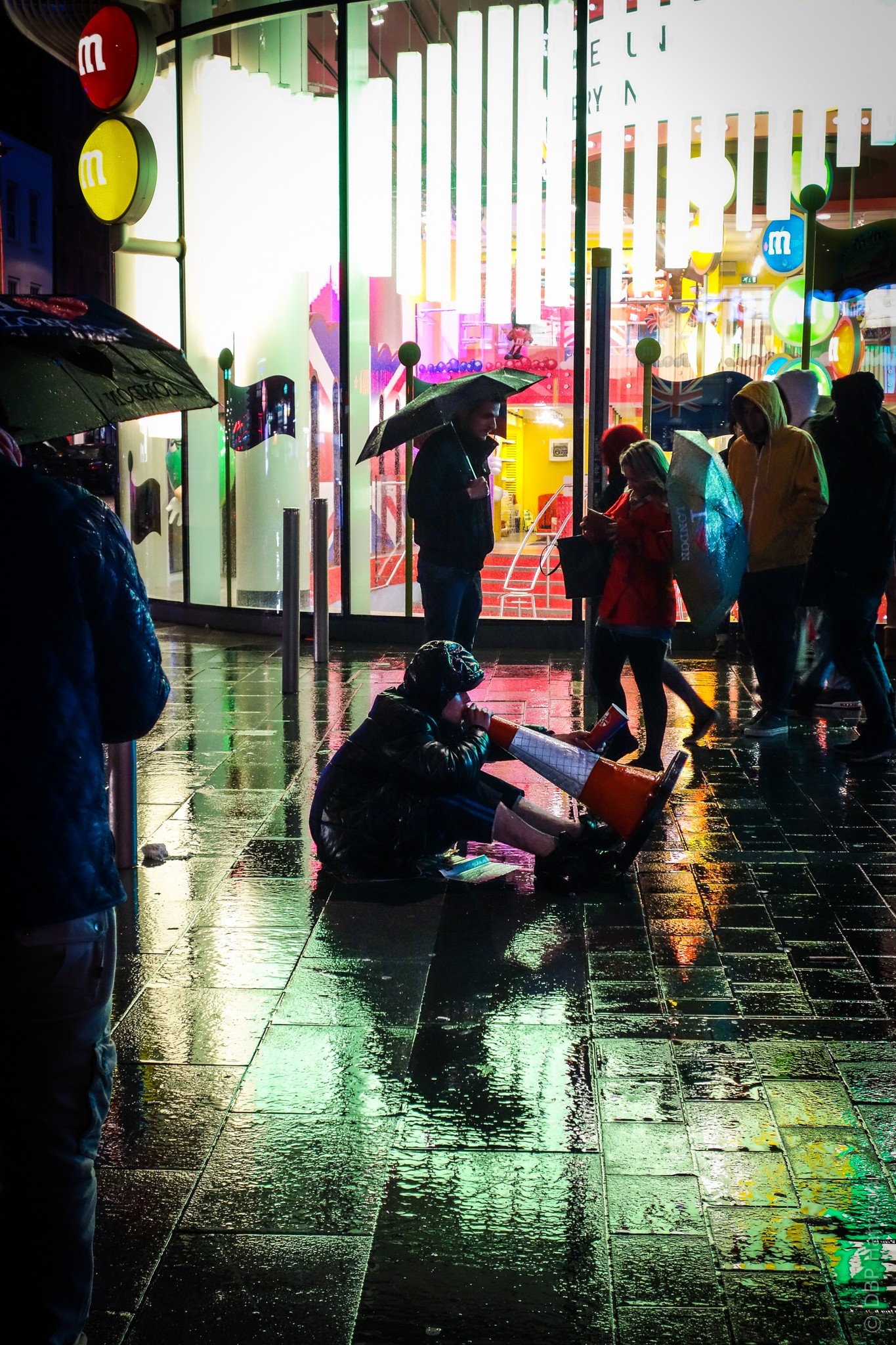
[831,730,896,762]
[626,759,665,774]
[559,823,625,853]
[683,707,719,744]
[599,735,639,761]
[533,830,620,886]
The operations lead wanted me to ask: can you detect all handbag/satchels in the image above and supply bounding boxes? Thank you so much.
[557,535,614,598]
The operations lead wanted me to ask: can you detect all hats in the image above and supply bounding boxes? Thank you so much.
[600,425,643,467]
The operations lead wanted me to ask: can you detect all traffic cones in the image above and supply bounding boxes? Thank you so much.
[483,716,689,873]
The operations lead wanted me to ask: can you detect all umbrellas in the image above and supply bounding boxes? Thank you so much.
[0,290,220,445]
[356,366,548,481]
[655,429,750,637]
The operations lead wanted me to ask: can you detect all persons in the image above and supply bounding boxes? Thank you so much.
[579,425,720,770]
[0,433,169,1345]
[722,369,896,764]
[408,396,501,655]
[309,639,622,880]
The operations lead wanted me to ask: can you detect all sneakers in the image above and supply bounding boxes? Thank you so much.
[813,687,862,709]
[744,712,789,737]
[739,710,765,730]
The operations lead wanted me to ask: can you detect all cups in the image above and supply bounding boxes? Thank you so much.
[583,703,630,752]
